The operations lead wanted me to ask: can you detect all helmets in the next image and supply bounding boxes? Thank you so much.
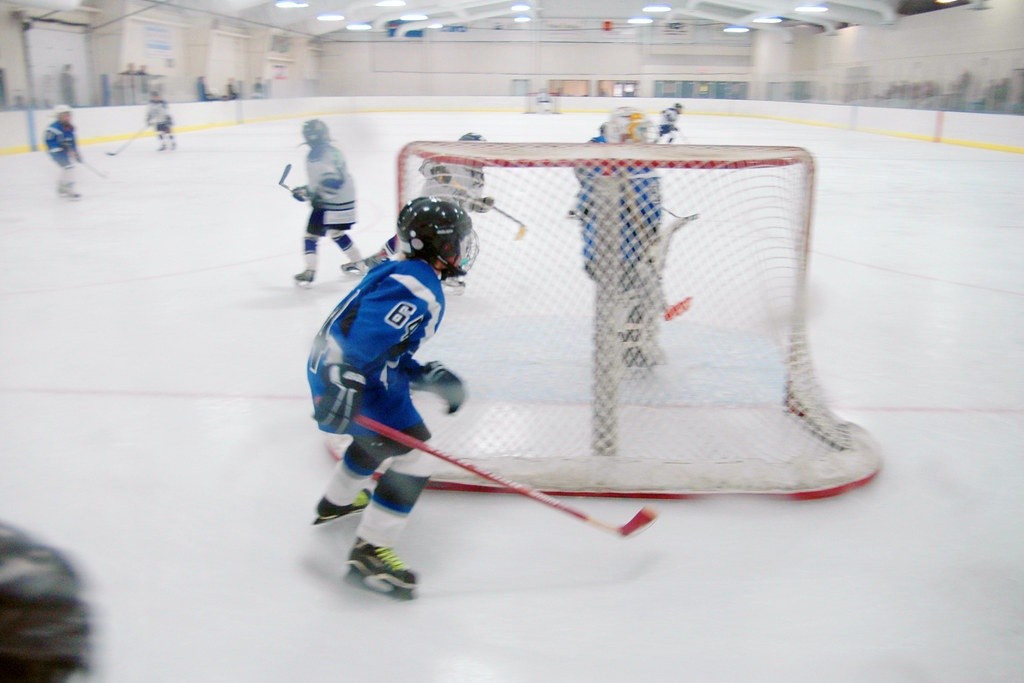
[303,120,328,149]
[1,533,89,669]
[606,109,651,146]
[397,196,471,258]
[459,131,486,143]
[54,103,71,117]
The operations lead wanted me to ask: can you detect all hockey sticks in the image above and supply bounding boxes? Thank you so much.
[312,395,658,537]
[75,157,105,177]
[439,176,526,240]
[279,164,307,201]
[619,167,691,321]
[106,123,150,156]
[661,206,699,220]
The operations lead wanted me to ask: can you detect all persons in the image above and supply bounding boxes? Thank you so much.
[566,106,663,368]
[0,519,95,683]
[652,102,684,143]
[60,63,75,106]
[44,104,83,198]
[291,119,365,281]
[306,196,480,590]
[115,63,167,104]
[197,75,211,102]
[146,90,177,150]
[419,132,488,287]
[224,76,240,100]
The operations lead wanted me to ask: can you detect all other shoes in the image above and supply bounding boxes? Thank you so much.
[60,189,68,195]
[170,144,174,149]
[66,190,78,198]
[159,145,166,152]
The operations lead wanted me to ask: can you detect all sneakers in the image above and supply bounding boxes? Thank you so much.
[295,271,315,287]
[342,261,364,281]
[315,488,372,527]
[343,539,416,600]
[442,278,465,296]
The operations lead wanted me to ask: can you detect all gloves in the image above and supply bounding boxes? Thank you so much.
[314,365,366,434]
[474,195,497,213]
[293,187,311,202]
[452,187,474,207]
[419,362,464,414]
